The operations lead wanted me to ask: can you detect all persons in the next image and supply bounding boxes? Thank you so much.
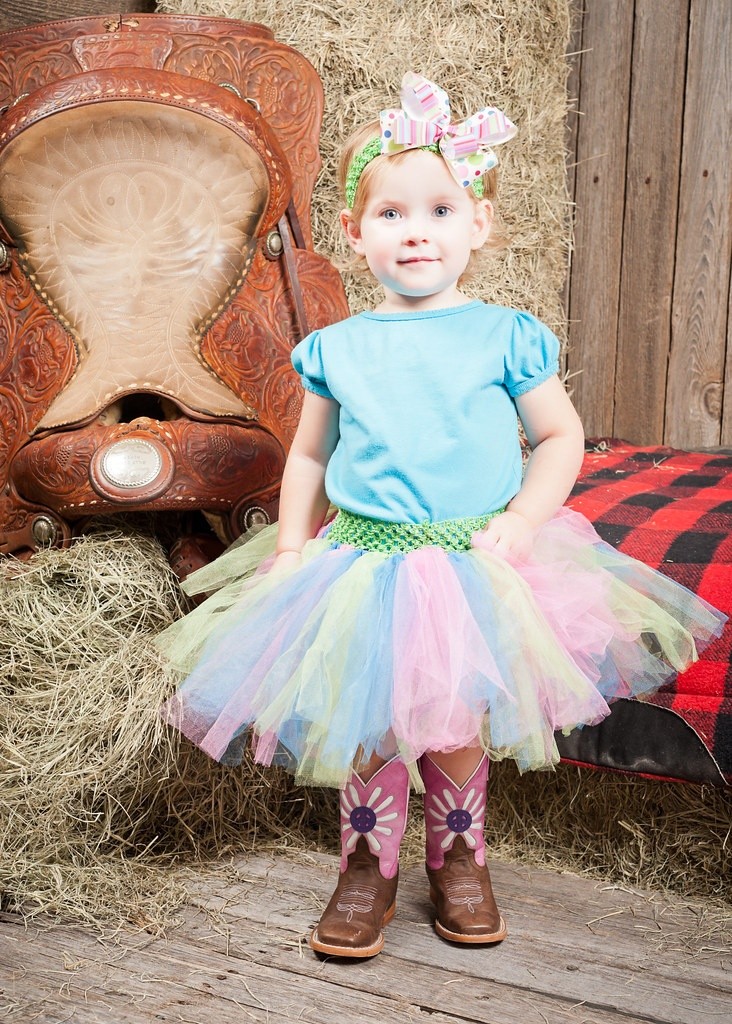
[155,72,728,957]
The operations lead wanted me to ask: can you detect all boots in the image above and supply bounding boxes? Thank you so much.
[309,753,411,959]
[421,750,509,944]
